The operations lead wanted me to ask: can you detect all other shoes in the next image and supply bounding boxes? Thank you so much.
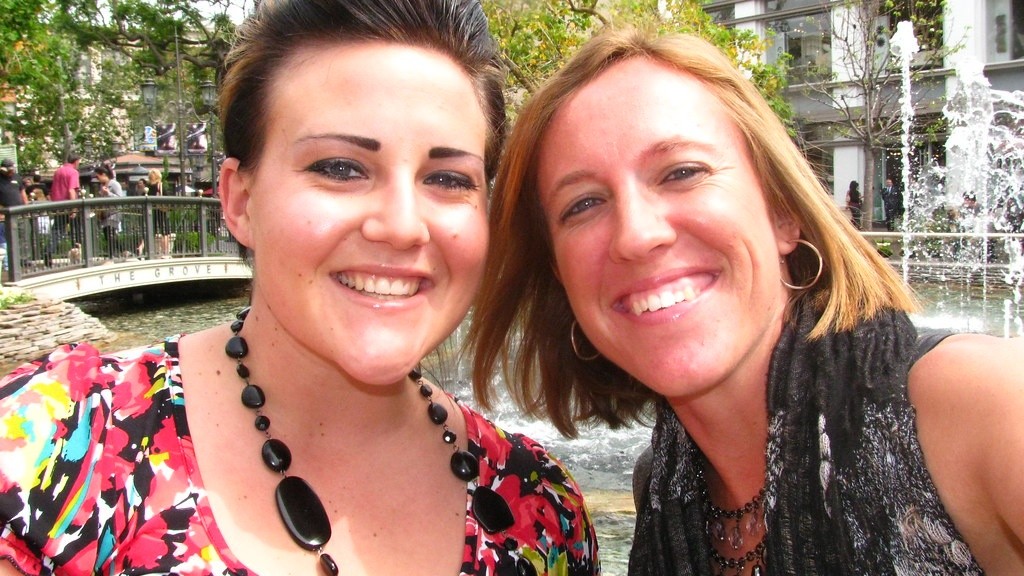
[859,226,864,231]
[44,252,52,268]
[136,248,142,261]
[102,259,113,265]
[161,255,172,259]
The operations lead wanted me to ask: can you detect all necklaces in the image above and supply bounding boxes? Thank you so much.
[227,306,537,576]
[693,445,769,576]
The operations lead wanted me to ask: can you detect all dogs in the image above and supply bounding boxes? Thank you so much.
[68,243,81,261]
[138,233,176,261]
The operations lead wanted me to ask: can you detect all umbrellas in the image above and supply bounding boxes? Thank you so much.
[169,183,220,195]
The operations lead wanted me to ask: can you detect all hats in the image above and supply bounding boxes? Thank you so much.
[68,153,81,163]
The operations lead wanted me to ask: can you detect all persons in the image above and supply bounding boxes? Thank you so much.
[846,181,863,230]
[881,179,899,232]
[943,190,1024,263]
[455,18,1024,576]
[0,0,602,576]
[1,153,172,284]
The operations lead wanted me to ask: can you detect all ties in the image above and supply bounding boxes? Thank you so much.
[888,189,889,194]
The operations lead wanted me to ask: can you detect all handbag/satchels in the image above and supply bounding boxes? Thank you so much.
[859,199,866,212]
